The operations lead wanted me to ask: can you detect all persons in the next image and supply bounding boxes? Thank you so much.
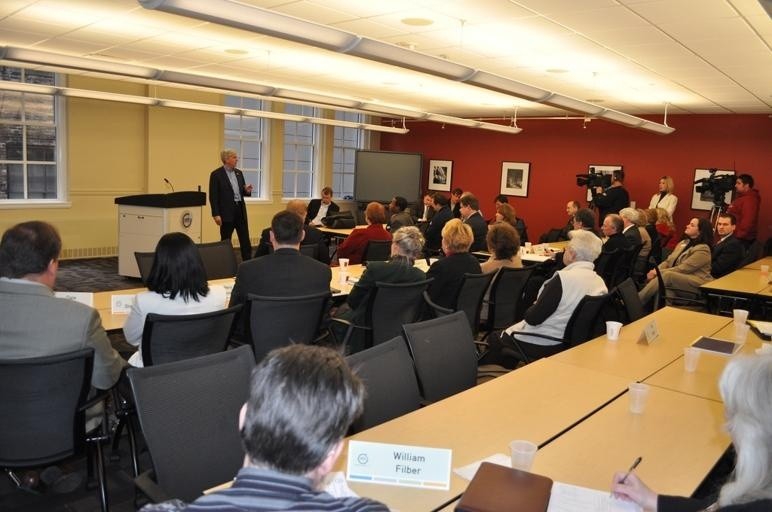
[308,187,528,266]
[540,170,759,277]
[335,225,429,342]
[480,220,525,329]
[117,232,228,402]
[209,148,253,267]
[621,217,717,316]
[256,198,331,266]
[0,221,125,499]
[610,345,772,511]
[497,228,608,367]
[426,217,484,318]
[137,345,389,511]
[230,209,333,349]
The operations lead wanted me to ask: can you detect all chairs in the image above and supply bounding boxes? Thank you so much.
[1,347,113,512]
[124,345,258,508]
[228,220,700,361]
[110,305,241,480]
[344,335,425,439]
[477,286,621,369]
[401,310,518,402]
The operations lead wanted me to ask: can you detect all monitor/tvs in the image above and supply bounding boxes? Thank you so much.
[353,150,422,206]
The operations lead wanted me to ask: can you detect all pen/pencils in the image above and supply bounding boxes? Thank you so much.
[612,457,642,497]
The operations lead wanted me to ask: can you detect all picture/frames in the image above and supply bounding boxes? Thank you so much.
[499,162,530,197]
[428,160,453,191]
[586,165,622,201]
[691,168,737,210]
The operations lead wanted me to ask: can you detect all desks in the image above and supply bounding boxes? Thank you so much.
[548,306,733,383]
[202,358,639,512]
[642,319,772,403]
[437,382,733,511]
[698,253,772,322]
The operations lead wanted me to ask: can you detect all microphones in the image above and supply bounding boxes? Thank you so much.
[164,178,174,193]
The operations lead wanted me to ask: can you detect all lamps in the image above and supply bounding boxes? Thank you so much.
[0,80,409,134]
[154,70,274,96]
[342,36,474,82]
[538,92,677,136]
[461,69,551,103]
[268,87,361,110]
[139,1,358,53]
[1,46,159,80]
[356,103,523,134]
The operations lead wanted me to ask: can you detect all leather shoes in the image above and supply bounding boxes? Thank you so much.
[19,470,41,495]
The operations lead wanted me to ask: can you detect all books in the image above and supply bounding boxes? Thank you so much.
[688,336,745,355]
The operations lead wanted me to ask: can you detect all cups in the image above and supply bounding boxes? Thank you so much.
[339,272,352,286]
[733,309,749,327]
[628,382,649,413]
[509,440,537,473]
[525,242,531,255]
[683,346,701,371]
[339,258,349,270]
[760,264,769,281]
[735,323,750,344]
[605,321,623,343]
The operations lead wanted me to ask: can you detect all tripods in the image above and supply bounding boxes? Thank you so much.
[709,203,728,239]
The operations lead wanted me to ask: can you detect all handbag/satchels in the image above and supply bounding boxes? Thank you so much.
[326,210,356,228]
